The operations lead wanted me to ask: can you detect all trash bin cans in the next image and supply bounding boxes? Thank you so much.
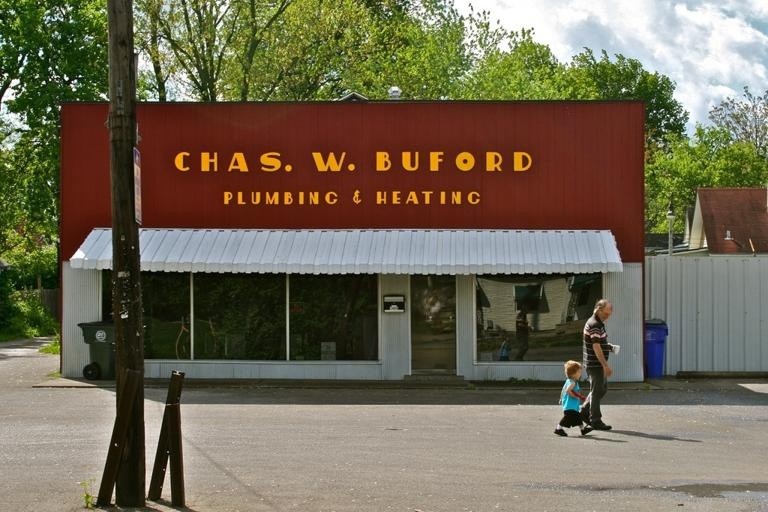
[644,318,668,378]
[78,321,117,380]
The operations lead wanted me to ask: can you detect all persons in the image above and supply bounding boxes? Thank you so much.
[553,360,594,436]
[577,298,612,430]
[499,337,512,361]
[514,303,531,361]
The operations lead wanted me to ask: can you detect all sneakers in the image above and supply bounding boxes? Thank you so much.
[579,407,612,431]
[553,428,568,436]
[578,427,594,437]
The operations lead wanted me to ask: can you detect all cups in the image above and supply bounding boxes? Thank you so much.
[613,344,621,356]
